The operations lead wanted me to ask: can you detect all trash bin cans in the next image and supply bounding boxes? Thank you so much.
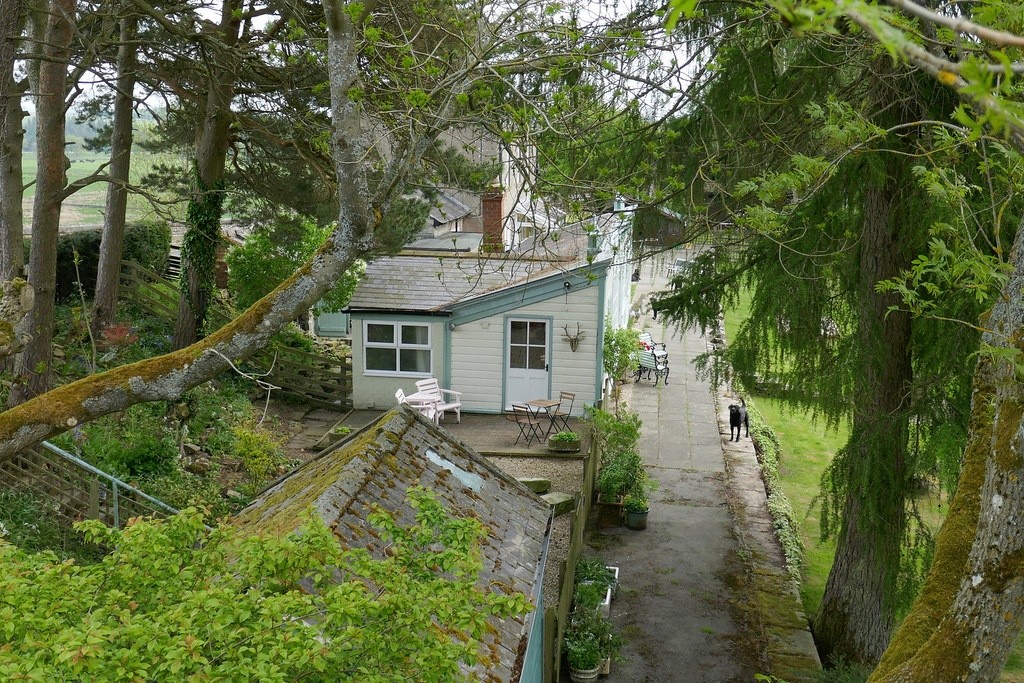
[683,243,692,250]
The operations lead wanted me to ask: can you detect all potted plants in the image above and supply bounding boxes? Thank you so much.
[560,554,638,683]
[547,429,583,452]
[583,326,661,532]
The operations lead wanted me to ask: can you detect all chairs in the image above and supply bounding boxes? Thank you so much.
[394,387,436,422]
[511,403,543,447]
[638,332,668,366]
[549,391,578,435]
[635,349,670,387]
[416,379,463,424]
[666,263,677,278]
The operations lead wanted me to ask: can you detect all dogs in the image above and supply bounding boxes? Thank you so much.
[727,398,749,442]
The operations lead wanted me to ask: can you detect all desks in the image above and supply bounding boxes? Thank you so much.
[408,391,441,421]
[676,259,695,275]
[526,398,563,439]
